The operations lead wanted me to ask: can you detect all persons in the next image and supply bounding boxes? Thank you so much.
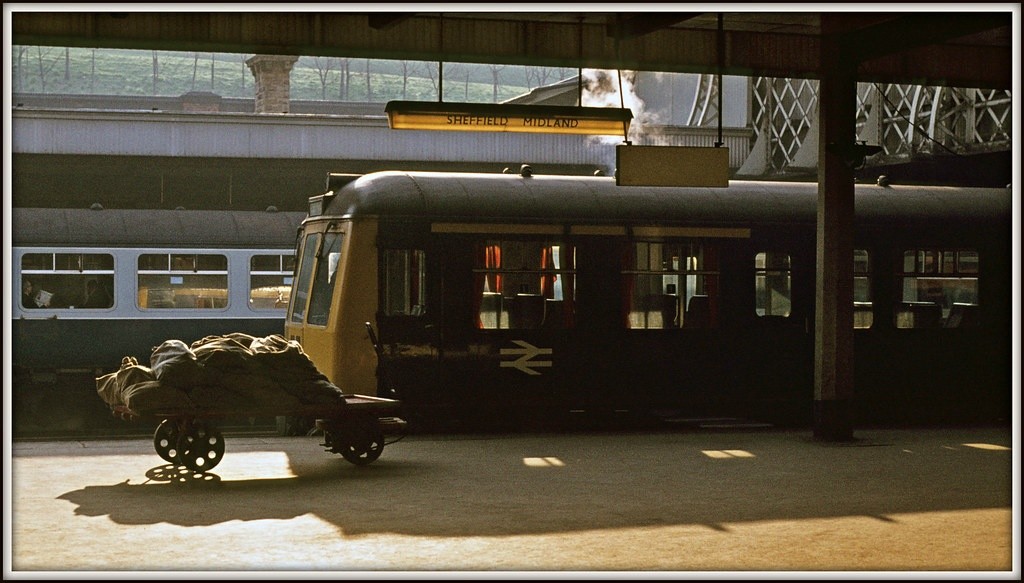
[22,279,51,308]
[86,279,108,308]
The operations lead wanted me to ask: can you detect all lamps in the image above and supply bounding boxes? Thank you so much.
[384,100,633,135]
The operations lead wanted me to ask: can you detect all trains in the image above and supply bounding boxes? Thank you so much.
[12,208,312,420]
[282,163,1014,425]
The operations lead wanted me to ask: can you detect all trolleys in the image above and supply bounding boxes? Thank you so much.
[109,321,408,474]
